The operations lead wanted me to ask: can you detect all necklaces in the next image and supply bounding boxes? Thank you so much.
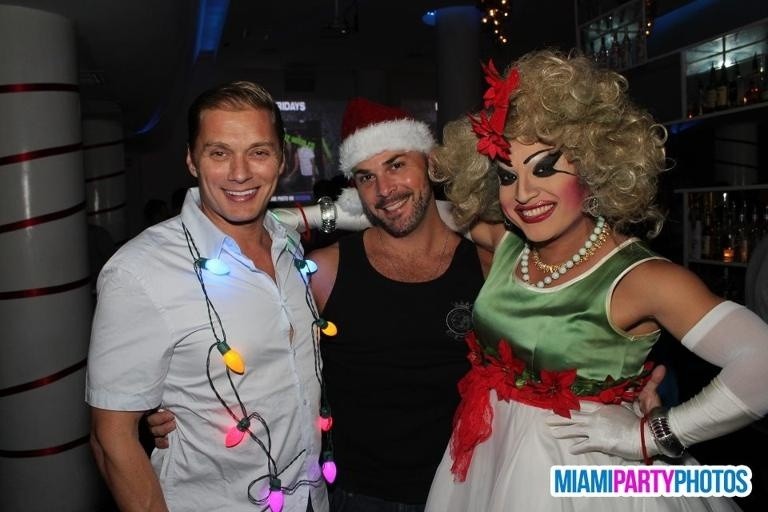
[520,216,612,289]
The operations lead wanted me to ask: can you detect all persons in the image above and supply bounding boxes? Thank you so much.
[295,141,317,191]
[87,78,334,512]
[269,51,768,511]
[279,138,300,192]
[146,97,669,510]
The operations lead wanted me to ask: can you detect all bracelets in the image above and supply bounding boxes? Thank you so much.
[317,196,338,236]
[294,202,311,242]
[640,405,694,468]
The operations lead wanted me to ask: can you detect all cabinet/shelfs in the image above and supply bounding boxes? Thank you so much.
[674,185,767,268]
[573,1,767,128]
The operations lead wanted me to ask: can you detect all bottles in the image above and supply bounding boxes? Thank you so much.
[585,20,766,266]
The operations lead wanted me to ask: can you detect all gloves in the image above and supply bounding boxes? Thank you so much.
[271,204,370,230]
[548,376,762,460]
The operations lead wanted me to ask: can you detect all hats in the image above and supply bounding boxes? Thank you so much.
[339,100,435,177]
